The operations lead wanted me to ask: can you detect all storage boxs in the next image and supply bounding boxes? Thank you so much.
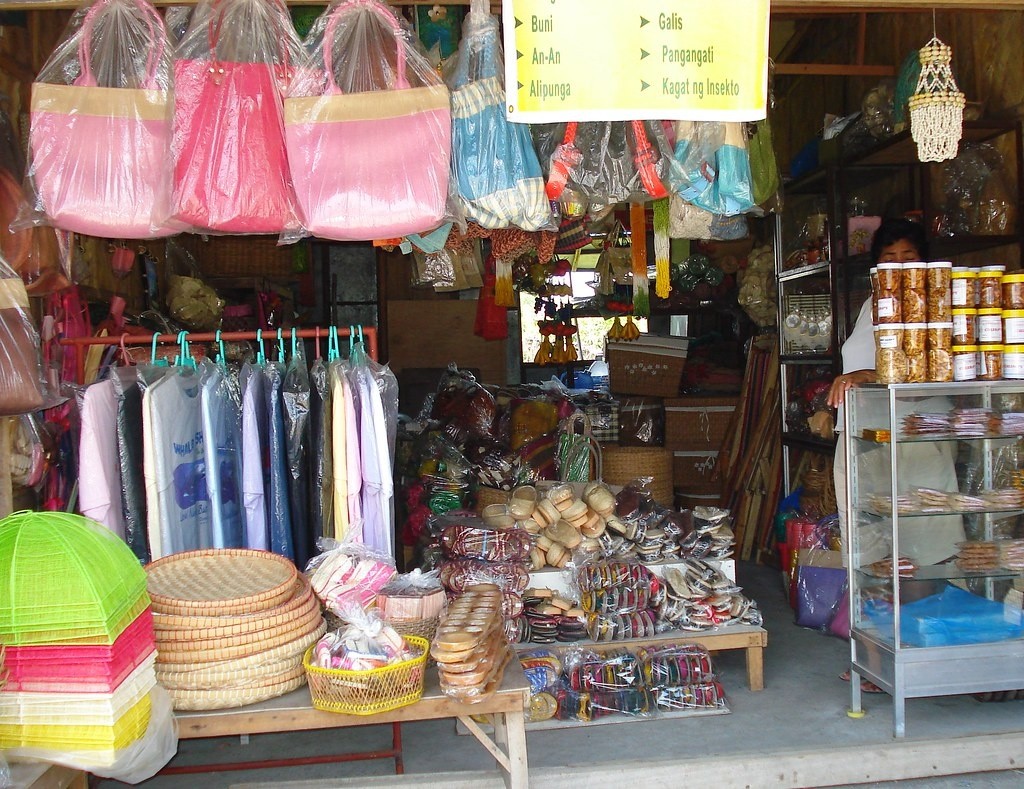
[596,443,676,510]
[664,396,740,453]
[672,451,730,497]
[672,491,730,538]
[618,396,664,447]
[608,335,690,398]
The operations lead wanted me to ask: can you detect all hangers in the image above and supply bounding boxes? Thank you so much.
[121,323,368,376]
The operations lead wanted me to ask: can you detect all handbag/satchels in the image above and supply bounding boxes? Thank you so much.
[670,115,782,242]
[284,1,452,242]
[443,17,553,234]
[28,0,188,241]
[478,223,640,287]
[171,0,314,233]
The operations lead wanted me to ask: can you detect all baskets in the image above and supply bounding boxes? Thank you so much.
[303,636,429,716]
[144,549,328,710]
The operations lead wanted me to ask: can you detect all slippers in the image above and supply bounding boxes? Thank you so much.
[441,481,763,723]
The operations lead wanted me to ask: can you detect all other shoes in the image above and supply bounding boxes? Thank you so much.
[839,670,853,680]
[861,681,886,693]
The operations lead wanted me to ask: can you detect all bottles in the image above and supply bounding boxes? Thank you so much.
[589,355,611,393]
[870,261,1024,383]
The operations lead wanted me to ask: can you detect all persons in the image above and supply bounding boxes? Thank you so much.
[823,218,969,693]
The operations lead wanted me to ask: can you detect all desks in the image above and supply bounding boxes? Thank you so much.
[0,643,532,789]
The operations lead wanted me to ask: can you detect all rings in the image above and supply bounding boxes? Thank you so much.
[840,381,846,384]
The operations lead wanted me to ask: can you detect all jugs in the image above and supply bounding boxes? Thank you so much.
[561,370,594,390]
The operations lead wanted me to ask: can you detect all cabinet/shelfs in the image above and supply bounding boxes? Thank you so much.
[513,557,768,692]
[775,115,1024,741]
